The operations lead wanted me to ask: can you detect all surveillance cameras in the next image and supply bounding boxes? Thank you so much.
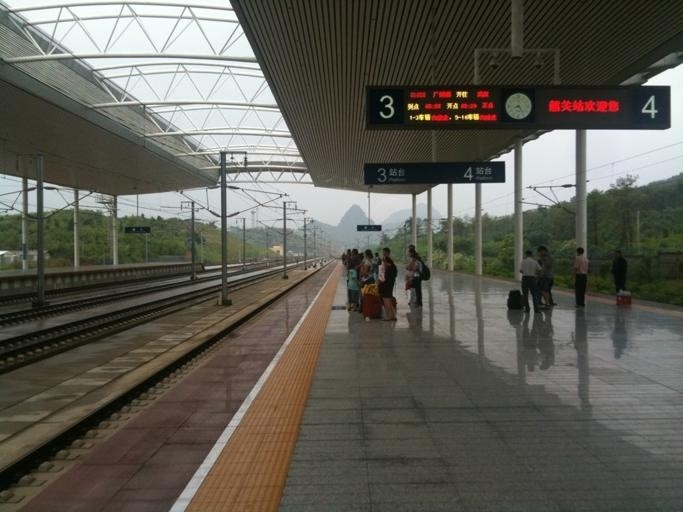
[486,59,501,70]
[535,61,546,70]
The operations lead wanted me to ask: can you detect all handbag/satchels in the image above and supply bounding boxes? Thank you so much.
[360,284,379,296]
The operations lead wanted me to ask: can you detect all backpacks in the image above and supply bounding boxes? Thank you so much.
[414,259,429,281]
[507,290,521,309]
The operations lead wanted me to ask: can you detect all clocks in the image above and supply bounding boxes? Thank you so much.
[504,93,533,120]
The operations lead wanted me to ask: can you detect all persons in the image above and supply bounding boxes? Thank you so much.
[574,247,589,307]
[523,306,555,372]
[342,245,423,321]
[611,310,627,359]
[520,246,558,313]
[614,251,627,293]
[571,309,588,364]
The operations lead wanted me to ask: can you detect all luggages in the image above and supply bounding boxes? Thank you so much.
[362,293,382,319]
